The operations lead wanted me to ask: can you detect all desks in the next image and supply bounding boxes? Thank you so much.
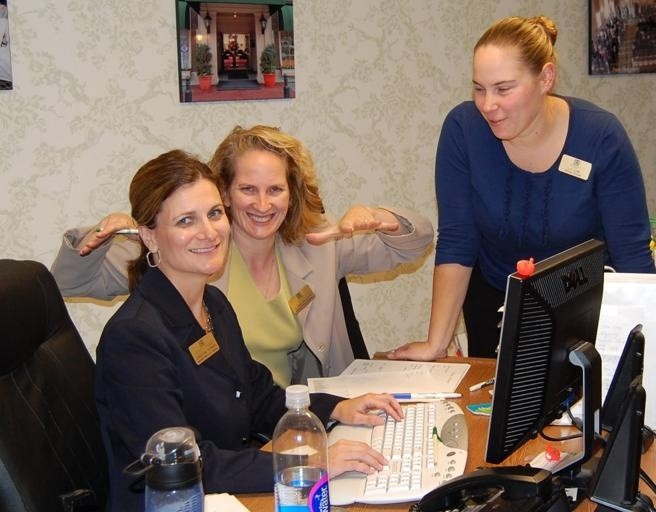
[231,352,655,512]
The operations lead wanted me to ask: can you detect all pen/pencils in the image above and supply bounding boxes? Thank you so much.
[95,227,139,235]
[469,378,494,392]
[389,391,463,400]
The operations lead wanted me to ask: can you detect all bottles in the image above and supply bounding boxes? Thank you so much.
[271,384,332,512]
[123,427,205,511]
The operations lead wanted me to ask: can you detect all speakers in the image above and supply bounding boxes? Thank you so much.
[600,324,654,452]
[588,382,653,511]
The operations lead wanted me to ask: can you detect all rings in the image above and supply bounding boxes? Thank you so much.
[391,350,396,353]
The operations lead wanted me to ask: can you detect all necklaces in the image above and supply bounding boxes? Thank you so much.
[202,299,214,333]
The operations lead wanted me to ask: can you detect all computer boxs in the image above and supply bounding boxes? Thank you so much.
[548,272,655,434]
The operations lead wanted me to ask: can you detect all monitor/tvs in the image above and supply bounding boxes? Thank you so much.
[485,239,606,463]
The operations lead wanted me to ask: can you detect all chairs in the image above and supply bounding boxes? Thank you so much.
[0,259,109,512]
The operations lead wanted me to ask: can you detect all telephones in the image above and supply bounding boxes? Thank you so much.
[409,463,561,512]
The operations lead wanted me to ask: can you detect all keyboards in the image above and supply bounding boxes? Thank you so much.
[325,400,468,507]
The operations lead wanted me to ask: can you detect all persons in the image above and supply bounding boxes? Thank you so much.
[91,149,405,512]
[368,17,656,362]
[47,125,434,388]
